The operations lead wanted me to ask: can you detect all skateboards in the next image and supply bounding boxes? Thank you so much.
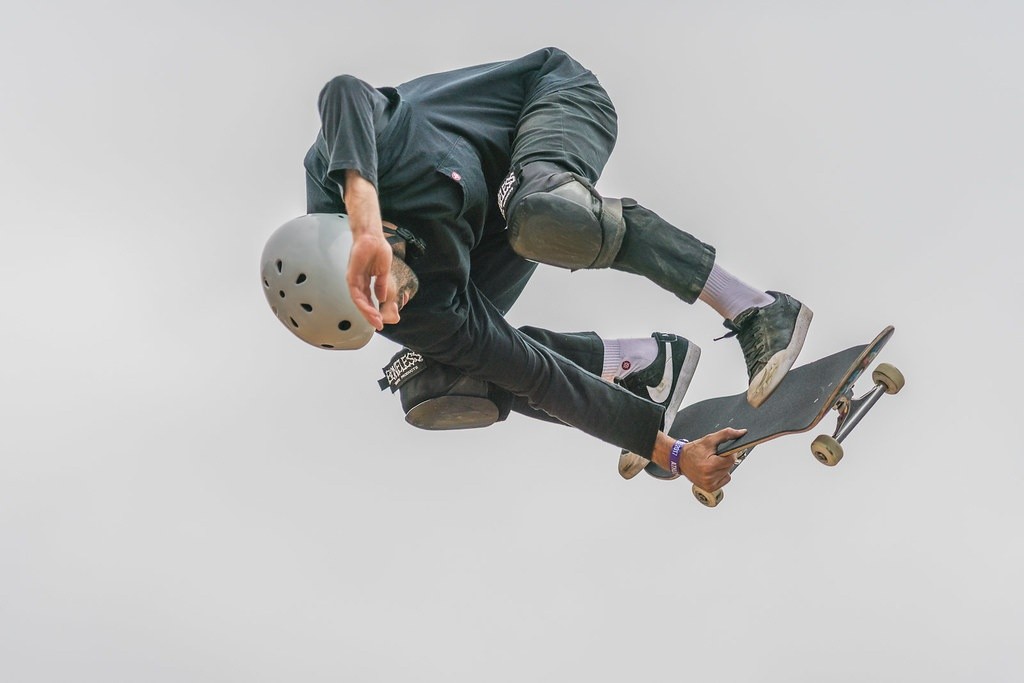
[643,324,904,510]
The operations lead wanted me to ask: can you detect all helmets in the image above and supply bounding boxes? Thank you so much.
[260,212,379,350]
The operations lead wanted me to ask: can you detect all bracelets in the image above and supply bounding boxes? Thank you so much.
[669,439,689,476]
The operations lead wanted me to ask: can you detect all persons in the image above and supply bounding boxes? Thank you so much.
[260,48,813,493]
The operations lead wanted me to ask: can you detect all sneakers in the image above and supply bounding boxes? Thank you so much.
[612,331,700,480]
[713,291,814,409]
